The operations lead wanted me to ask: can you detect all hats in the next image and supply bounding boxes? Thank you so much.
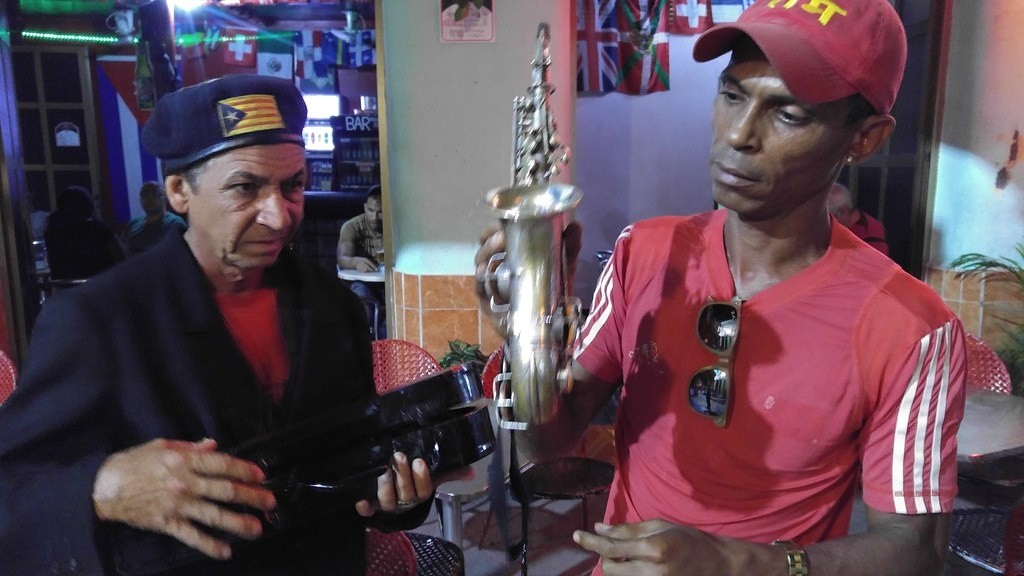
[140,74,307,177]
[693,0,907,116]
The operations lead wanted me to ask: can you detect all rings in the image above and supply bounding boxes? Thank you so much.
[396,498,417,510]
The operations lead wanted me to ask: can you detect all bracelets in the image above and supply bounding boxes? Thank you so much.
[775,540,809,576]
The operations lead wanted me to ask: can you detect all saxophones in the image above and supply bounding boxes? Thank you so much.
[482,24,587,468]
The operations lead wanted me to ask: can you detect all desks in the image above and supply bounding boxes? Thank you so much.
[955,384,1024,511]
[431,396,583,576]
[337,264,386,340]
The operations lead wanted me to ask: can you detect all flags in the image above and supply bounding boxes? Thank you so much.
[292,30,376,80]
[711,0,757,24]
[175,24,220,62]
[576,0,617,94]
[616,0,670,94]
[257,29,292,80]
[223,28,257,67]
[657,0,715,36]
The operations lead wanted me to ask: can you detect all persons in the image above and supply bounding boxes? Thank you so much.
[0,74,436,576]
[474,0,968,576]
[825,182,889,257]
[121,182,188,253]
[38,186,125,285]
[337,185,387,346]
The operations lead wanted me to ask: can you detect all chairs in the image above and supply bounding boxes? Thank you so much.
[363,337,506,576]
[942,330,1023,576]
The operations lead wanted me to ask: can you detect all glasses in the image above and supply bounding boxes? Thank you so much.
[687,297,747,427]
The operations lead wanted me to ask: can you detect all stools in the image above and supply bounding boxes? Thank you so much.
[513,457,615,576]
